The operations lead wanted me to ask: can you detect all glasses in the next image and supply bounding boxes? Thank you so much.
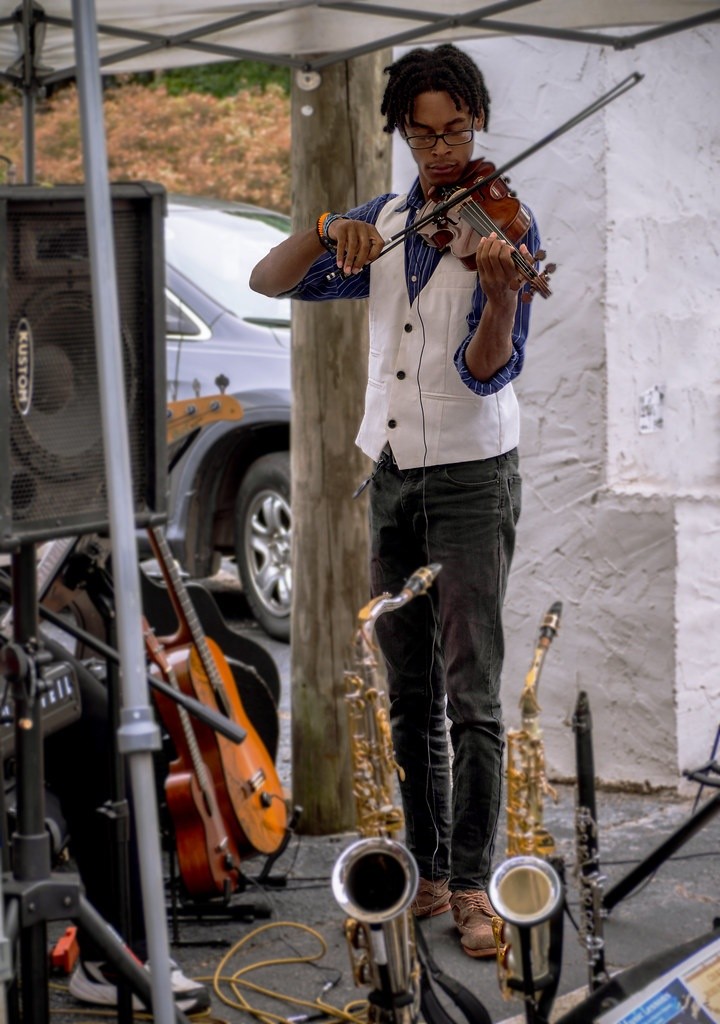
[402,112,476,149]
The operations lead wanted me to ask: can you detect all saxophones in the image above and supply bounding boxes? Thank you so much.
[487,600,569,1024]
[328,558,444,1023]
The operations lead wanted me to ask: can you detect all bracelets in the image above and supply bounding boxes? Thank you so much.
[317,209,351,245]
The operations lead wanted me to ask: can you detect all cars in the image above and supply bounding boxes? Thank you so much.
[138,196,295,641]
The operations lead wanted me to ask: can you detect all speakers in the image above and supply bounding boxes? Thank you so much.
[0,179,171,552]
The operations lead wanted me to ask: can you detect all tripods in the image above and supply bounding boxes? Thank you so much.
[0,539,193,1024]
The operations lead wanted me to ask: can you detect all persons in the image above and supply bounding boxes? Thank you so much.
[0,543,212,1018]
[249,43,540,958]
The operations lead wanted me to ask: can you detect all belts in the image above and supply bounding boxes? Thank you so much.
[382,443,398,465]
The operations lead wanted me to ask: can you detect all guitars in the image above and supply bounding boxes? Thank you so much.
[90,509,291,901]
[165,374,246,446]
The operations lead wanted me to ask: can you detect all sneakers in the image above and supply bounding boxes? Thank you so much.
[69,947,210,1015]
[409,876,452,921]
[449,888,503,956]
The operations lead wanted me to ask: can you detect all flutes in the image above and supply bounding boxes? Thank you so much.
[573,689,611,997]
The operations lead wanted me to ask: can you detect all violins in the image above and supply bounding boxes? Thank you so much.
[412,158,558,304]
